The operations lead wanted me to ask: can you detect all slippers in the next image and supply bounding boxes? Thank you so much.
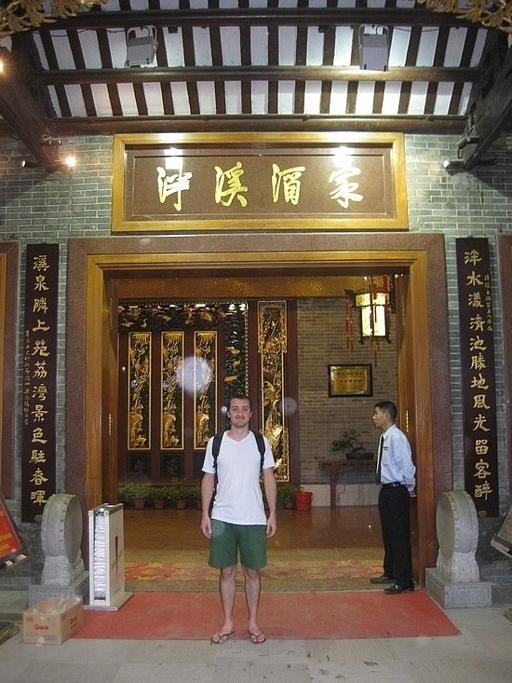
[210,630,236,643]
[247,629,266,644]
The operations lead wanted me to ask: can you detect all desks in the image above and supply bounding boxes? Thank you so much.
[327,458,378,509]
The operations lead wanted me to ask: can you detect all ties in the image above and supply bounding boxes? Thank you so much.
[375,436,384,487]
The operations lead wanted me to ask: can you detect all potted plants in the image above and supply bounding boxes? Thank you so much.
[276,482,302,510]
[124,479,202,513]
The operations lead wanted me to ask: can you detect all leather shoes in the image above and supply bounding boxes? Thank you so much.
[385,581,414,594]
[369,573,398,585]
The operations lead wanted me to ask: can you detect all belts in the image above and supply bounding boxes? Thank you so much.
[383,482,401,488]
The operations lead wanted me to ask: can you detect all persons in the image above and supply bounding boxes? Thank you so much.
[370,401,416,594]
[200,395,278,644]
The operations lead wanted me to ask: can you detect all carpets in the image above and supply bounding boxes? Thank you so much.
[77,588,463,641]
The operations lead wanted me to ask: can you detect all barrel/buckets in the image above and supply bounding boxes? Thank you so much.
[296,491,312,511]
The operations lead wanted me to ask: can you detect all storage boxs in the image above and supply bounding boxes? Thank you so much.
[22,594,85,648]
